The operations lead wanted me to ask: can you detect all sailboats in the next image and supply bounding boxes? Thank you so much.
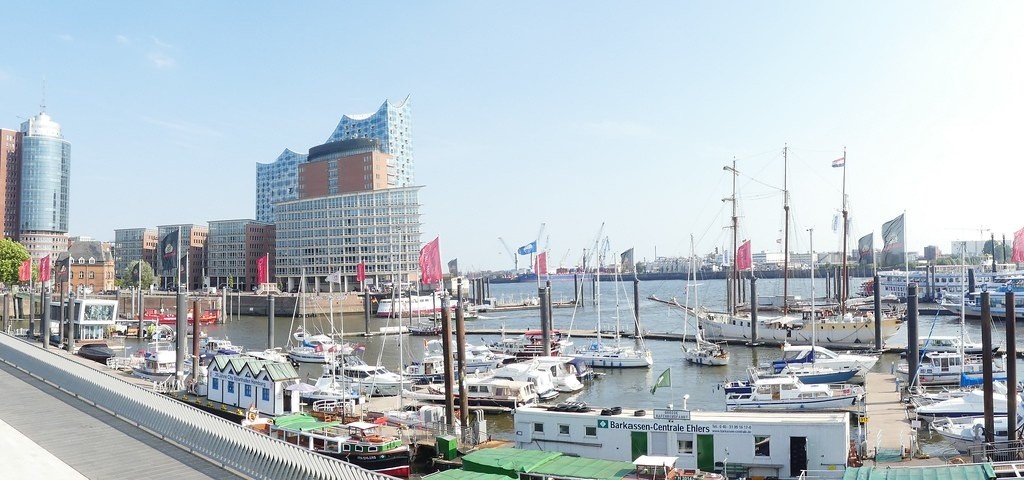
[560,219,654,366]
[678,231,731,367]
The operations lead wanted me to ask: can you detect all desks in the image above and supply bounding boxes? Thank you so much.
[632,455,680,480]
[348,421,379,436]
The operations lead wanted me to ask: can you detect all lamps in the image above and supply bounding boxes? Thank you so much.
[683,394,689,409]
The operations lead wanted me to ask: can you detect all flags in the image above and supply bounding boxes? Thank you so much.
[858,213,906,268]
[256,255,267,284]
[325,270,340,284]
[131,262,139,283]
[534,252,547,275]
[448,258,457,276]
[1010,226,1024,262]
[38,254,50,282]
[737,240,752,270]
[832,157,844,167]
[313,343,365,354]
[56,257,69,283]
[176,255,186,284]
[419,237,444,285]
[620,248,635,274]
[356,262,365,281]
[161,229,178,271]
[650,367,672,395]
[19,260,30,282]
[517,240,538,255]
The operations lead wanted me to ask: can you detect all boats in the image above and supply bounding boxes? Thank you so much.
[644,139,1024,455]
[38,216,607,479]
